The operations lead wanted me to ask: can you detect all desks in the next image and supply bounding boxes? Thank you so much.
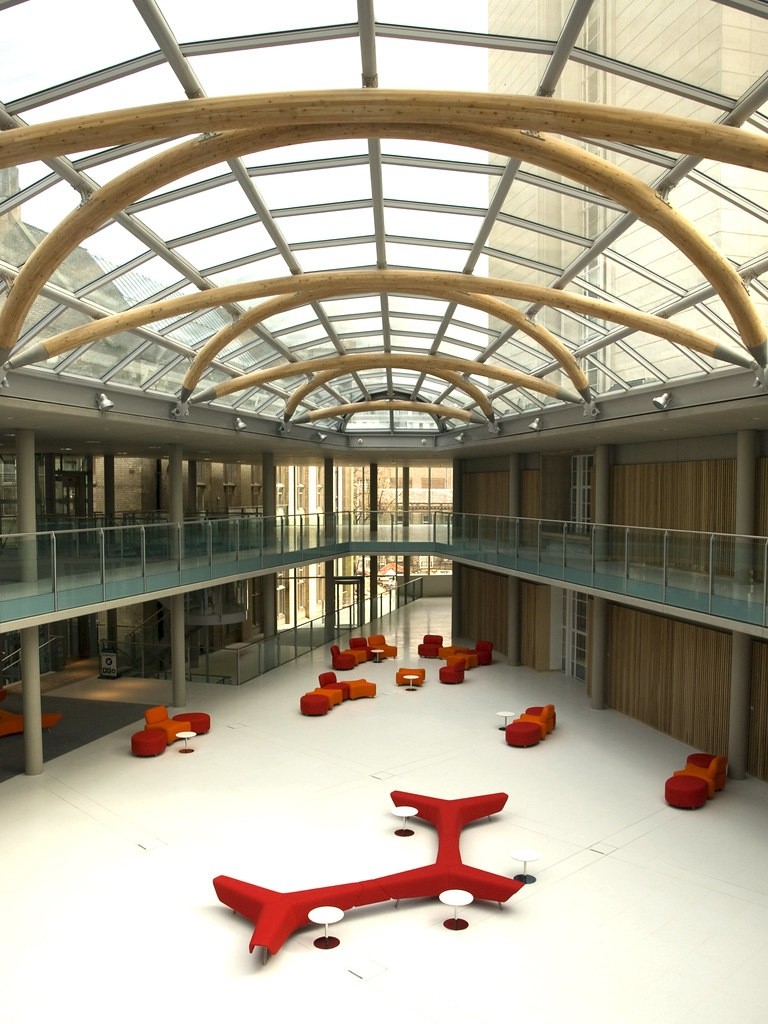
[438,889,474,931]
[391,805,419,836]
[403,675,419,691]
[513,851,537,884]
[371,649,384,662]
[497,712,516,731]
[308,906,344,948]
[176,731,197,753]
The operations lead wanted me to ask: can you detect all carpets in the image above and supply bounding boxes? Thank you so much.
[0,692,170,784]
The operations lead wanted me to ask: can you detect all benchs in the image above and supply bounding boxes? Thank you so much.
[301,632,495,716]
[213,790,532,964]
[0,686,62,739]
[506,704,556,748]
[665,752,728,809]
[131,705,211,757]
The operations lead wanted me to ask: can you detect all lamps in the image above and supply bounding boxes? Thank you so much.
[317,431,327,443]
[96,393,114,411]
[455,432,465,443]
[235,417,247,431]
[529,418,543,432]
[653,393,672,411]
